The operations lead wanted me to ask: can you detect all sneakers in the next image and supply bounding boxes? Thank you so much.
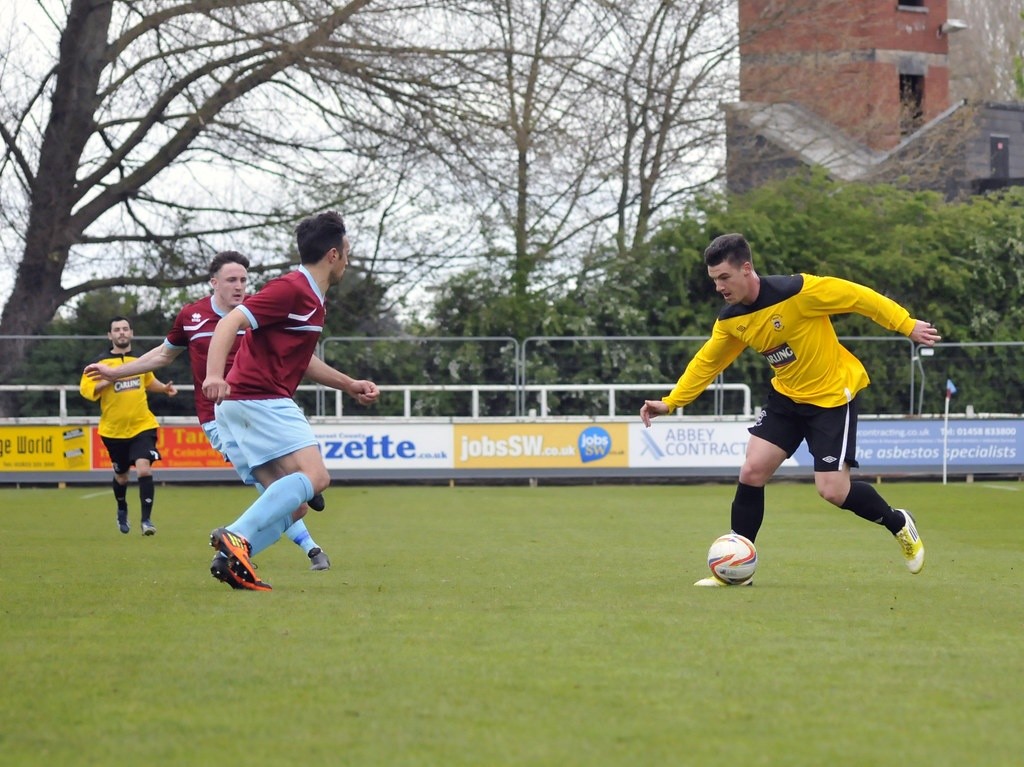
[208,526,262,583]
[118,516,130,534]
[694,575,752,588]
[210,551,272,592]
[893,508,925,574]
[311,553,331,571]
[307,492,325,511]
[141,520,156,536]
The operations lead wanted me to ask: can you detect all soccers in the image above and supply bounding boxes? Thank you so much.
[707,533,758,586]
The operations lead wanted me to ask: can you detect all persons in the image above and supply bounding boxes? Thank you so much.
[640,233,941,586]
[84,250,380,571]
[78,315,178,536]
[202,211,350,591]
